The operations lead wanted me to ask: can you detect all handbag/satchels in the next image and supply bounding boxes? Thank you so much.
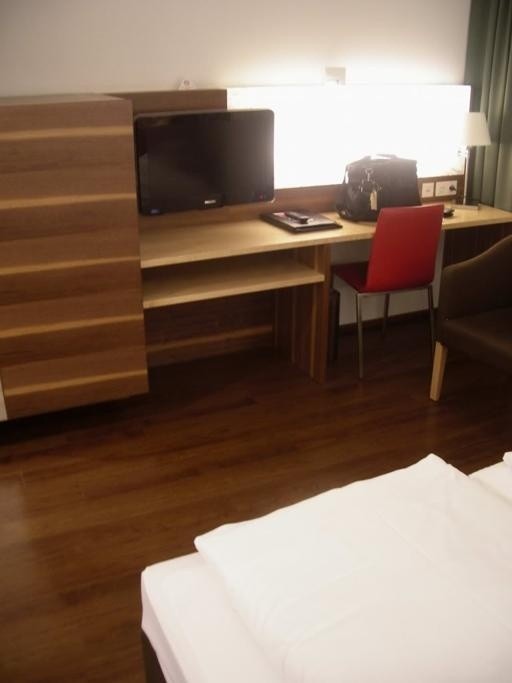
[337,154,421,222]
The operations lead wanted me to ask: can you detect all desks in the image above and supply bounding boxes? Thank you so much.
[141,197,512,387]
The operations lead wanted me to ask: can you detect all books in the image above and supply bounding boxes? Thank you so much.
[261,210,343,233]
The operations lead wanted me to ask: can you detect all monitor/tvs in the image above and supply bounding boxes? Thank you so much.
[133,109,276,215]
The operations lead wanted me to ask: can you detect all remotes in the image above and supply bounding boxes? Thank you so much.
[284,211,310,223]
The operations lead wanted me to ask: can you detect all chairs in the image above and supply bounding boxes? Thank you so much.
[328,202,512,401]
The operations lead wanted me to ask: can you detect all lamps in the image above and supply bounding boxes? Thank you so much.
[453,111,491,210]
